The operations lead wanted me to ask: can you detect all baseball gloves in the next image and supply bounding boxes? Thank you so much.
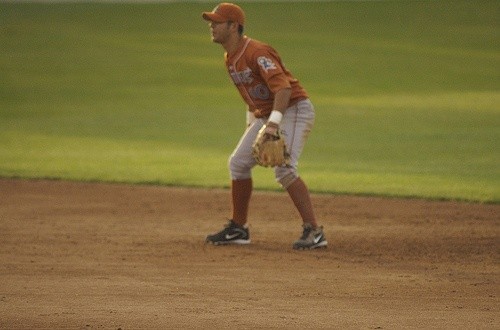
[249,123,292,169]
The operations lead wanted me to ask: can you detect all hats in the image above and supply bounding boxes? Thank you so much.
[202,3,245,26]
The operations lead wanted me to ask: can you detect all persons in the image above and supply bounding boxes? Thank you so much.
[202,3,329,250]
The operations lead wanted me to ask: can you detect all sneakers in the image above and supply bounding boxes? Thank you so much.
[206,220,251,246]
[291,223,327,250]
